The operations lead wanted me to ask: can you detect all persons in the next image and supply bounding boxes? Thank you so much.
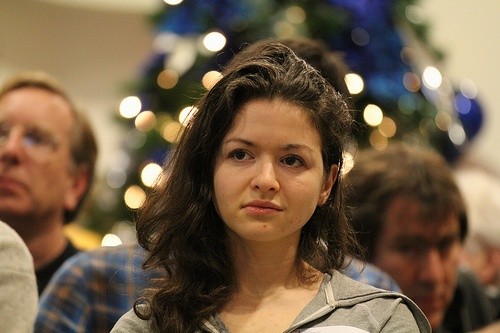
[339,141,500,332]
[32,37,403,333]
[110,42,432,333]
[0,75,98,300]
